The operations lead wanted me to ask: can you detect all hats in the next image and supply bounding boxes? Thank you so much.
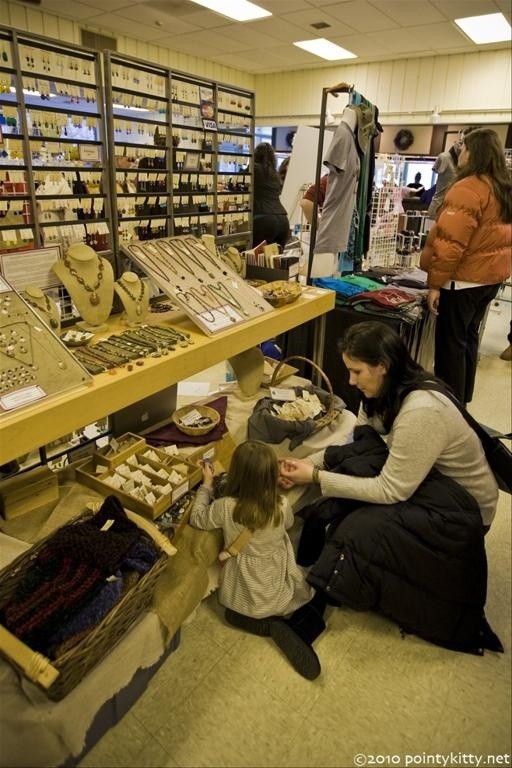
[345,103,379,156]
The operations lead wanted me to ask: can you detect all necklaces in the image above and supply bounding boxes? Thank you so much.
[129,235,267,327]
[31,331,69,371]
[24,295,60,330]
[74,324,197,376]
[1,318,40,373]
[115,279,146,316]
[63,253,108,307]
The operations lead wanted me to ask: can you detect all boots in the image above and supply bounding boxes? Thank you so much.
[223,606,278,636]
[269,607,326,681]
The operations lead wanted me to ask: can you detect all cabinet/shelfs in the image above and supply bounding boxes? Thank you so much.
[107,49,255,259]
[0,23,116,274]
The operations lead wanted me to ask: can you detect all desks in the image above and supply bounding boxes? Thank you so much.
[0,282,338,526]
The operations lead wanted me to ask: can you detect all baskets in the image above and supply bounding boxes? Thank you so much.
[259,356,343,437]
[0,499,176,705]
[172,405,221,436]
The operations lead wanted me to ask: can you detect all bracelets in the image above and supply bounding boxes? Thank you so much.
[313,464,321,488]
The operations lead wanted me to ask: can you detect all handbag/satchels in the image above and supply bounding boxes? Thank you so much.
[476,420,512,495]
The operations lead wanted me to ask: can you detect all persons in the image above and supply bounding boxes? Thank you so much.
[275,319,499,606]
[189,440,326,682]
[301,172,329,229]
[418,128,511,410]
[254,142,290,252]
[406,171,424,197]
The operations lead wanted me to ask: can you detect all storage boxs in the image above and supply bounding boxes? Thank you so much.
[1,462,60,521]
